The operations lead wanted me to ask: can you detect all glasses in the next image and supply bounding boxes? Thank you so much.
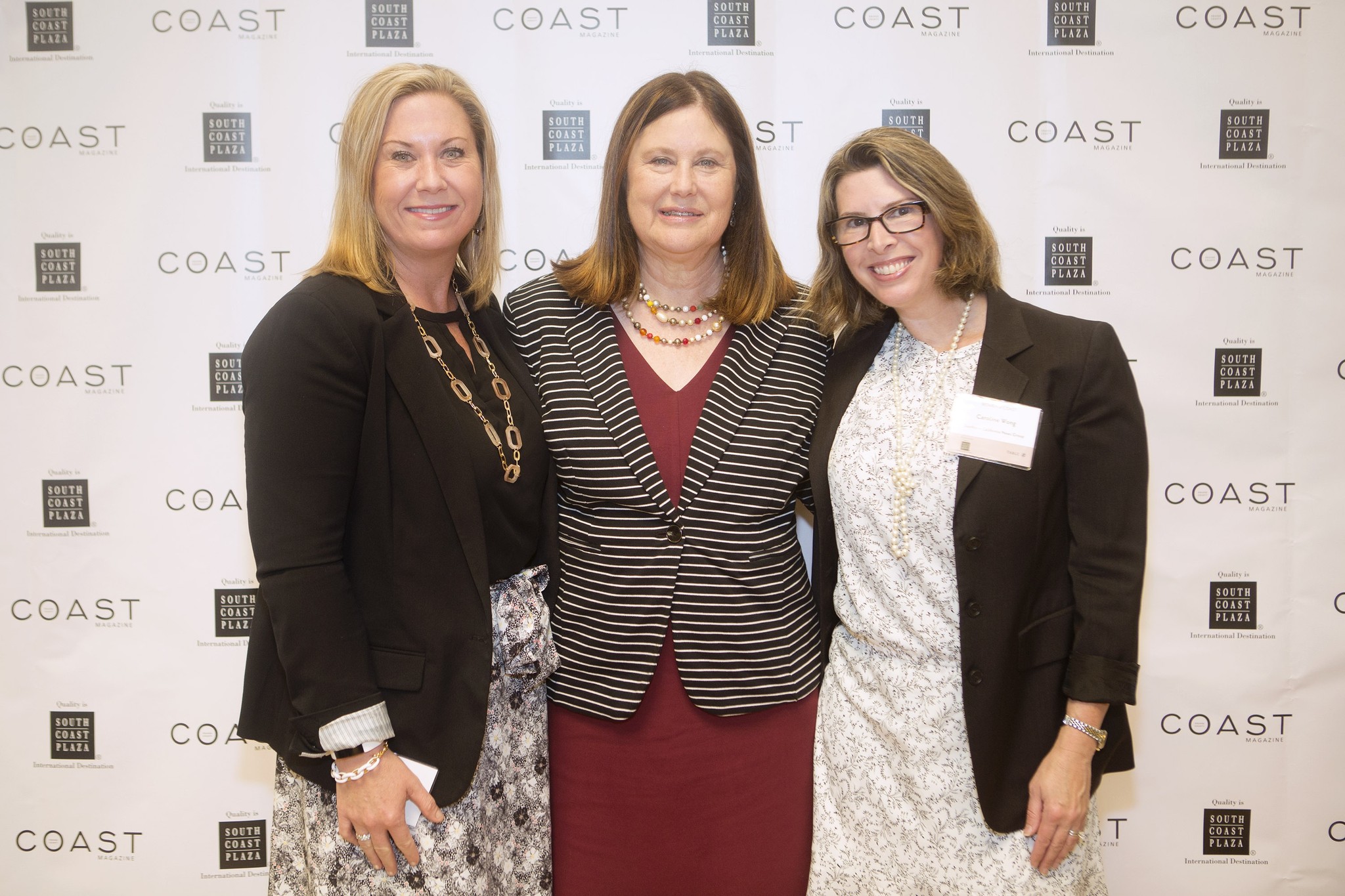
[824,201,931,247]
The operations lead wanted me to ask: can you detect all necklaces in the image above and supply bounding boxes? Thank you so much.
[622,241,736,347]
[408,274,522,483]
[891,289,977,561]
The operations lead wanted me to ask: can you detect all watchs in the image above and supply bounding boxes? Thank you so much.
[1062,714,1106,752]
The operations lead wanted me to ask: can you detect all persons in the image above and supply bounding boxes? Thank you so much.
[807,129,1150,896]
[236,64,561,896]
[501,71,834,896]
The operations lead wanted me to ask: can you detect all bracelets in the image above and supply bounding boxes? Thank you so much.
[331,742,384,760]
[331,741,389,784]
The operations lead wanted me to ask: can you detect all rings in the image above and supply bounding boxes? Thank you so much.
[355,833,371,841]
[1067,830,1088,839]
